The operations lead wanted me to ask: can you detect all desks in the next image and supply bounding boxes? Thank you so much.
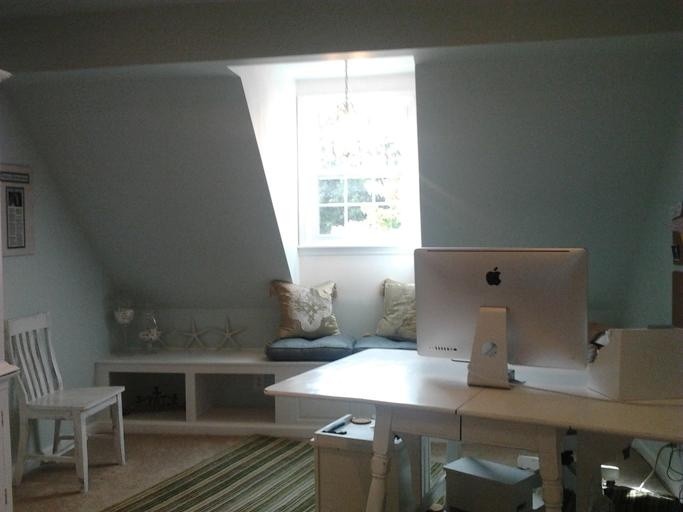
[261,349,682,510]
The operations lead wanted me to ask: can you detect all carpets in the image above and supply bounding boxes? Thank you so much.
[100,431,448,510]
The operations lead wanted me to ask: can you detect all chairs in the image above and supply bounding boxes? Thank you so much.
[4,309,126,496]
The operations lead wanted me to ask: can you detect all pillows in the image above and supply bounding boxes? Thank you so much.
[267,334,355,361]
[368,275,419,343]
[264,278,341,342]
[355,330,417,350]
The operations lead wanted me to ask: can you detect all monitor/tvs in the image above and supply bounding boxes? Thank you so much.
[414,247,588,389]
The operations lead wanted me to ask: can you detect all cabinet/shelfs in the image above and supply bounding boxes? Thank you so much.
[305,416,431,510]
[0,359,23,511]
